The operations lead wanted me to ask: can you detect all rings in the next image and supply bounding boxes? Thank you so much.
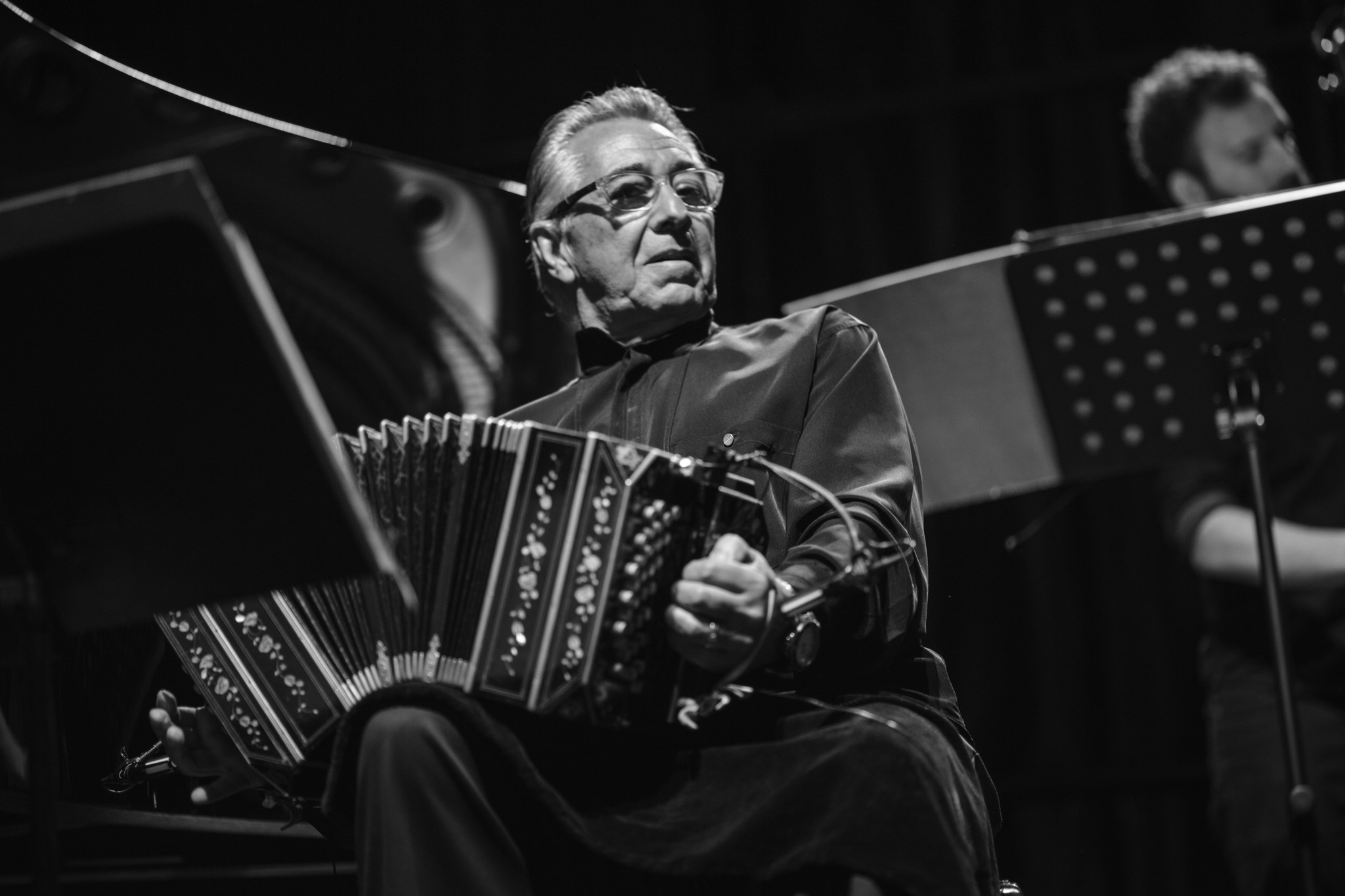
[704,621,721,650]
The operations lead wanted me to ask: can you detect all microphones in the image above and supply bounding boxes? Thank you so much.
[782,554,912,617]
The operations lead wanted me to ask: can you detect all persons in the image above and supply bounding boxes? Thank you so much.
[310,81,1020,896]
[1125,46,1345,896]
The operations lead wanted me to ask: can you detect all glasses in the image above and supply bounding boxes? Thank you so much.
[547,167,726,220]
